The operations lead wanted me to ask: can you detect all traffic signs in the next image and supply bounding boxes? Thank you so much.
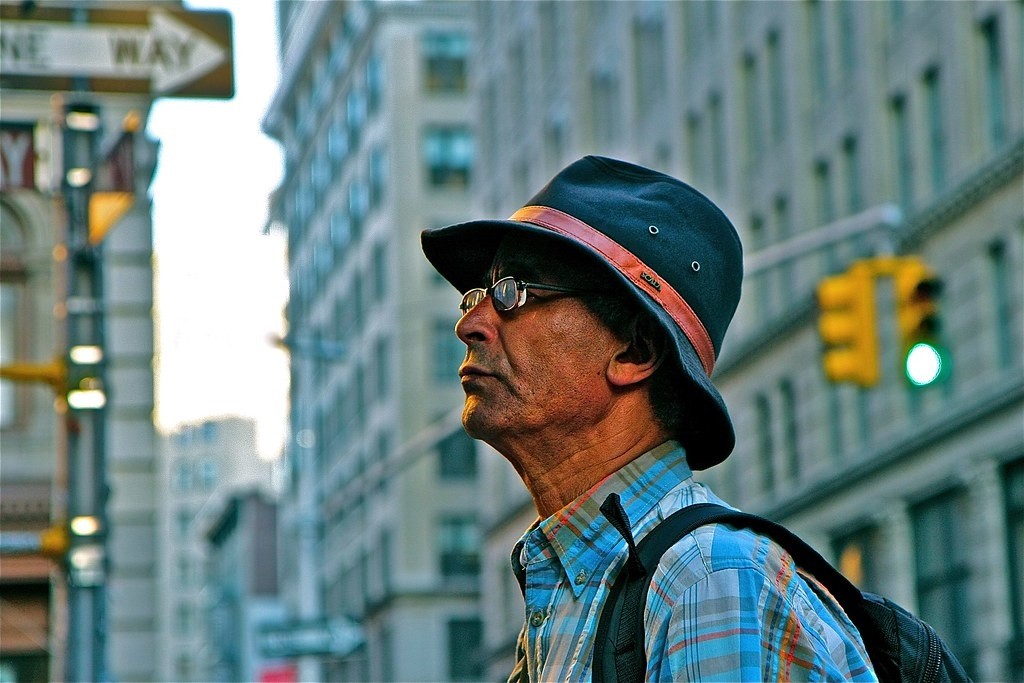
[250,613,369,661]
[0,2,235,104]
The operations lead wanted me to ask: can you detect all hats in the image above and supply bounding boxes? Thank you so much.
[421,155,744,471]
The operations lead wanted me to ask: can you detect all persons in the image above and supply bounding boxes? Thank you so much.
[420,155,880,683]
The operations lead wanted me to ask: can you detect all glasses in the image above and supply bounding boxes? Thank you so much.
[460,276,579,316]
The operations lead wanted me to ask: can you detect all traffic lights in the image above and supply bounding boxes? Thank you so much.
[892,253,950,385]
[815,267,878,391]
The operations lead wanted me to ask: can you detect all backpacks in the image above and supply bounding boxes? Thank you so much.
[590,493,974,683]
